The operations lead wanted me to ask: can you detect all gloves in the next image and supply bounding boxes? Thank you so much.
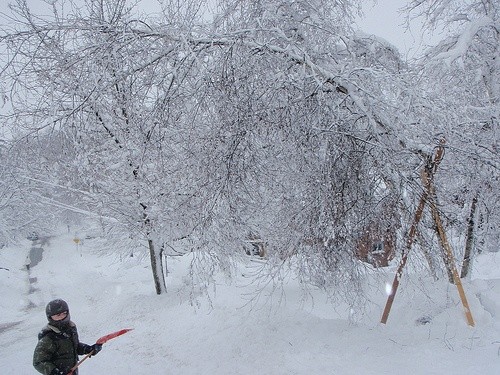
[88,344,102,358]
[58,368,77,375]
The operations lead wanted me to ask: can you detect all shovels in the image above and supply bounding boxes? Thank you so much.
[67,328,134,375]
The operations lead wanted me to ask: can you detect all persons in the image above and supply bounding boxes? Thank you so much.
[33,299,103,375]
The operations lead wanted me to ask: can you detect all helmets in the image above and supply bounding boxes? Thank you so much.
[46,299,70,327]
